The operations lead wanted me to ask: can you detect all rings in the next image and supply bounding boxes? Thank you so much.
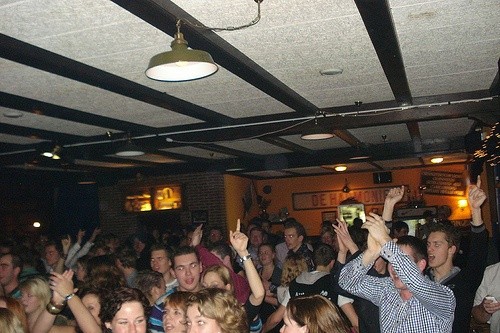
[53,283,56,286]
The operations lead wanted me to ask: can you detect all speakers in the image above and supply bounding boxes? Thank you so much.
[373,171,392,183]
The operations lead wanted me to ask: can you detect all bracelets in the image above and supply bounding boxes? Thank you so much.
[50,297,66,308]
[65,294,75,303]
[241,255,251,262]
[47,303,62,315]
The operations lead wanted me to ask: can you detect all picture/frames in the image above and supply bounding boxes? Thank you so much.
[192,210,208,224]
[322,211,337,222]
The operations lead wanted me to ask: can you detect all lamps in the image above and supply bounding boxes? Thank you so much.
[115,138,146,156]
[301,118,334,140]
[77,173,97,185]
[146,24,218,83]
[40,145,62,159]
[348,145,372,160]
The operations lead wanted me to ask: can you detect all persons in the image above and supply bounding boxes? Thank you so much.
[0,176,500,333]
